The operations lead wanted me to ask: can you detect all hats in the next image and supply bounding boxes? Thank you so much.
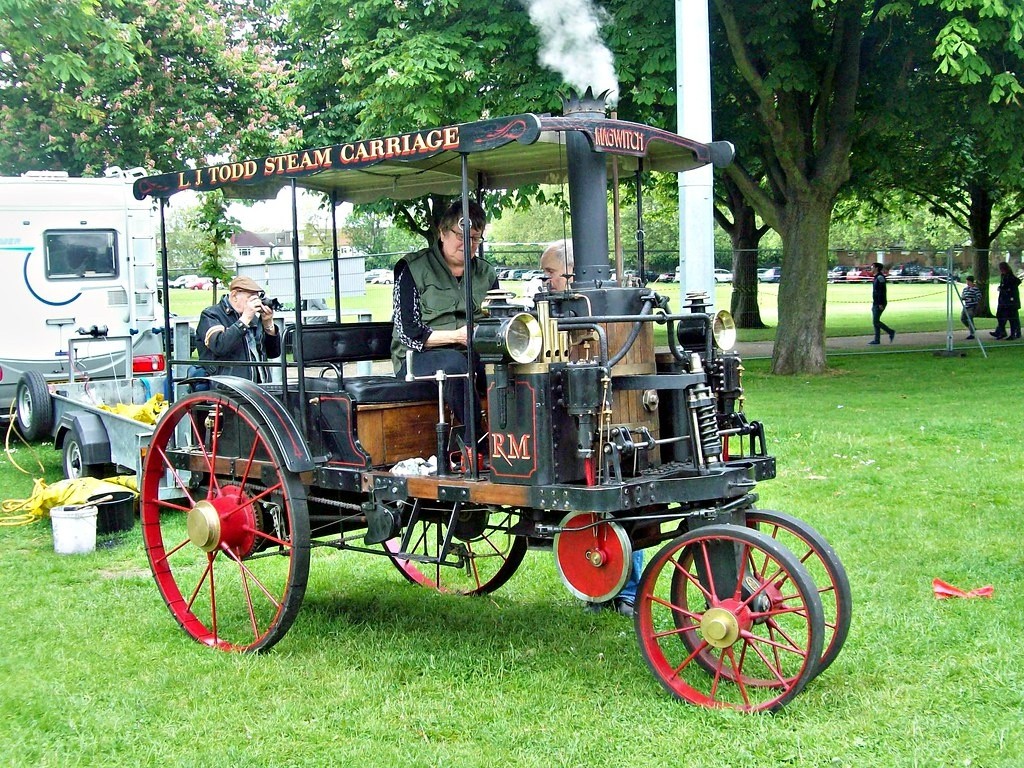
[229,275,263,292]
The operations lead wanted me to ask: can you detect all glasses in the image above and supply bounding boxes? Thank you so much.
[446,226,484,244]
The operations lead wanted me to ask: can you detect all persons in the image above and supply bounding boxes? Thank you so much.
[193,277,283,386]
[539,239,644,616]
[988,261,1022,341]
[960,276,982,339]
[389,197,508,474]
[869,263,896,345]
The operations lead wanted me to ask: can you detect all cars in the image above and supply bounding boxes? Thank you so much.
[202,281,222,289]
[658,272,673,282]
[188,278,211,290]
[494,267,544,281]
[714,268,734,284]
[610,265,659,283]
[846,263,888,284]
[827,266,849,281]
[156,276,168,288]
[372,272,397,285]
[218,277,236,290]
[365,267,378,283]
[919,266,961,283]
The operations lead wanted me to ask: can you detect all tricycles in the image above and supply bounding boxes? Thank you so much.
[131,116,852,718]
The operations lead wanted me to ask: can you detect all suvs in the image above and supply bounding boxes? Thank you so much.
[888,261,921,284]
[756,268,772,283]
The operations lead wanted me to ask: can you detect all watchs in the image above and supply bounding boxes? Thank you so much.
[264,324,274,331]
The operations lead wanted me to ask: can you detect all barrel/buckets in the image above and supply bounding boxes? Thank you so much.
[87,491,135,534]
[49,505,98,554]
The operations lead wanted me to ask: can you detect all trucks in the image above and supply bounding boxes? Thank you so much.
[171,274,198,288]
[0,164,371,503]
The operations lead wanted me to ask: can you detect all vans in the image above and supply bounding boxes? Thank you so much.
[674,266,681,282]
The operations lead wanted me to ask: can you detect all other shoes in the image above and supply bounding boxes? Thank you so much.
[889,329,896,343]
[989,331,1008,340]
[866,341,880,346]
[967,335,975,340]
[1006,331,1022,341]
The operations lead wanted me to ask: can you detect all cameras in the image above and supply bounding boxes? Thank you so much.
[256,293,283,312]
